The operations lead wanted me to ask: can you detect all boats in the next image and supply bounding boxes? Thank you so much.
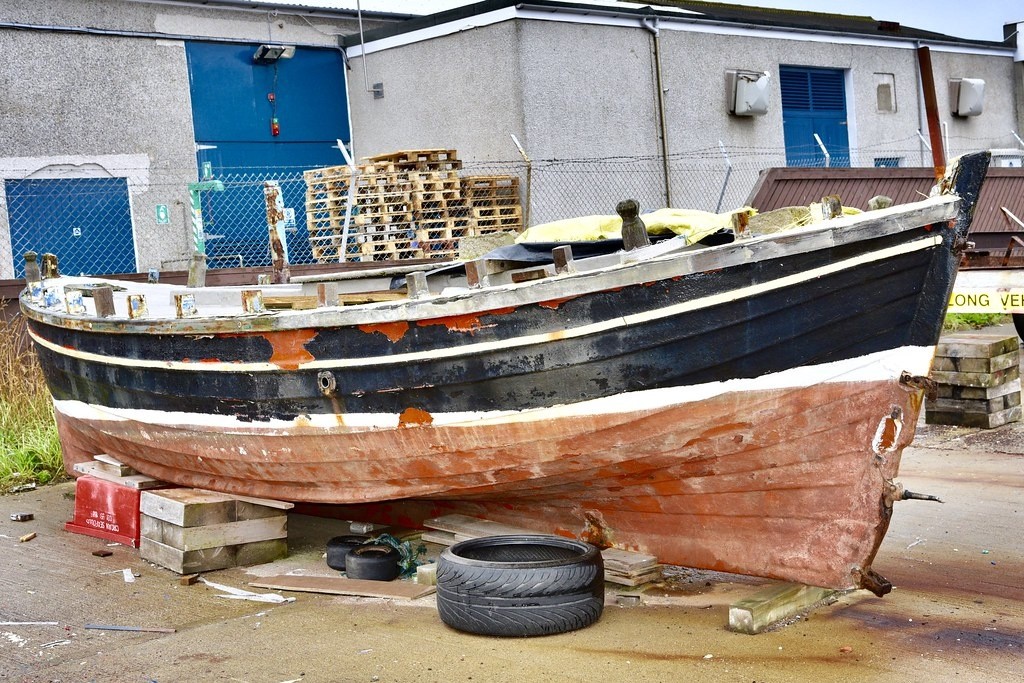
[14,148,998,592]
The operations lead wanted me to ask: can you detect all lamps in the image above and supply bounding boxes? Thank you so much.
[253,45,286,65]
